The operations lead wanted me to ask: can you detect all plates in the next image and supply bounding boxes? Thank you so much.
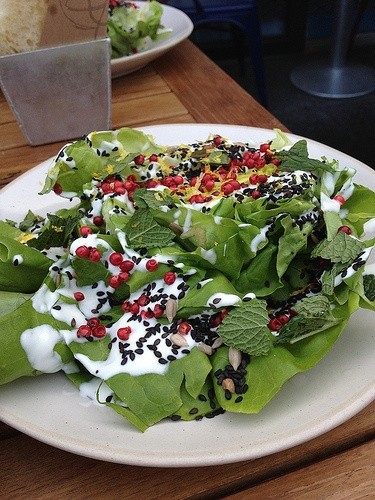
[0,124,375,468]
[105,0,194,80]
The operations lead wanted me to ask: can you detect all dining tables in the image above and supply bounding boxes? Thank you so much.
[0,38,375,500]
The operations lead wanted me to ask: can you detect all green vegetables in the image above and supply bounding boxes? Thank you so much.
[103,1,164,58]
[0,127,375,431]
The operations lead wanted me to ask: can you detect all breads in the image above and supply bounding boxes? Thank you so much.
[0,0,109,56]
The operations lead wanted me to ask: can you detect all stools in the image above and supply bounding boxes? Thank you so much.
[168,0,269,107]
